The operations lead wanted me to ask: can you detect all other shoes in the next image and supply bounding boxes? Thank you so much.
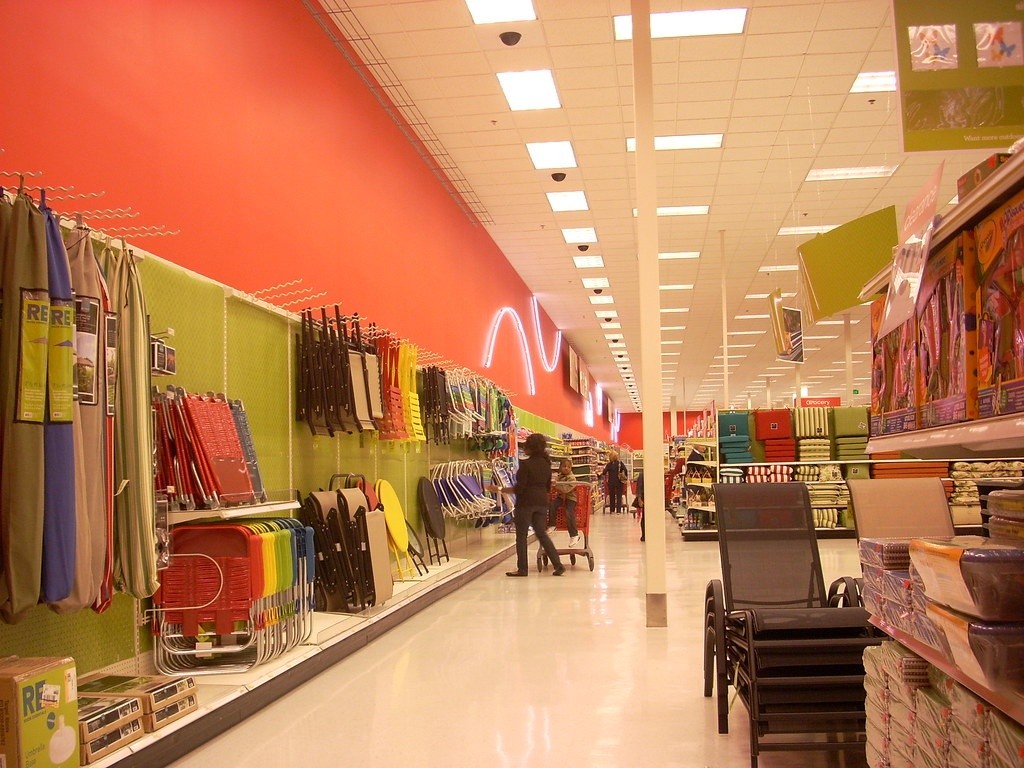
[553,566,566,576]
[640,537,644,541]
[617,512,620,515]
[506,569,526,576]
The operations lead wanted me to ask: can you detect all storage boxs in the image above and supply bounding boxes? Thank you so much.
[0,654,200,767]
[151,336,177,376]
[859,190,1024,767]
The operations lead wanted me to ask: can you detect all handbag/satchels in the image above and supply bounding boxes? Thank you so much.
[618,461,628,484]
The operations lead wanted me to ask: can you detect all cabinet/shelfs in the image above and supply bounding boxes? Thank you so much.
[519,437,718,541]
[722,458,1024,540]
[857,149,1024,726]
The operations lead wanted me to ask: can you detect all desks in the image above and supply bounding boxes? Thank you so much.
[375,476,450,582]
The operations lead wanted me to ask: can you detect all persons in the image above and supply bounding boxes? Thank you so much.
[505,433,566,577]
[632,469,645,541]
[688,444,706,461]
[545,459,581,547]
[602,450,628,515]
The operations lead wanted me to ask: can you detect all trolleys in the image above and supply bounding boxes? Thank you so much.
[603,473,629,514]
[537,481,594,572]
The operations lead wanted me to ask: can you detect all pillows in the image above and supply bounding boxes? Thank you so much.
[719,407,1024,529]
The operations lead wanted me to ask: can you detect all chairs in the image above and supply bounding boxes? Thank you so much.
[295,473,392,613]
[296,305,513,445]
[150,386,318,678]
[430,458,518,527]
[705,479,956,768]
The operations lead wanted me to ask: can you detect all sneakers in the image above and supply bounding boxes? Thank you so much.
[569,534,581,548]
[545,525,557,535]
